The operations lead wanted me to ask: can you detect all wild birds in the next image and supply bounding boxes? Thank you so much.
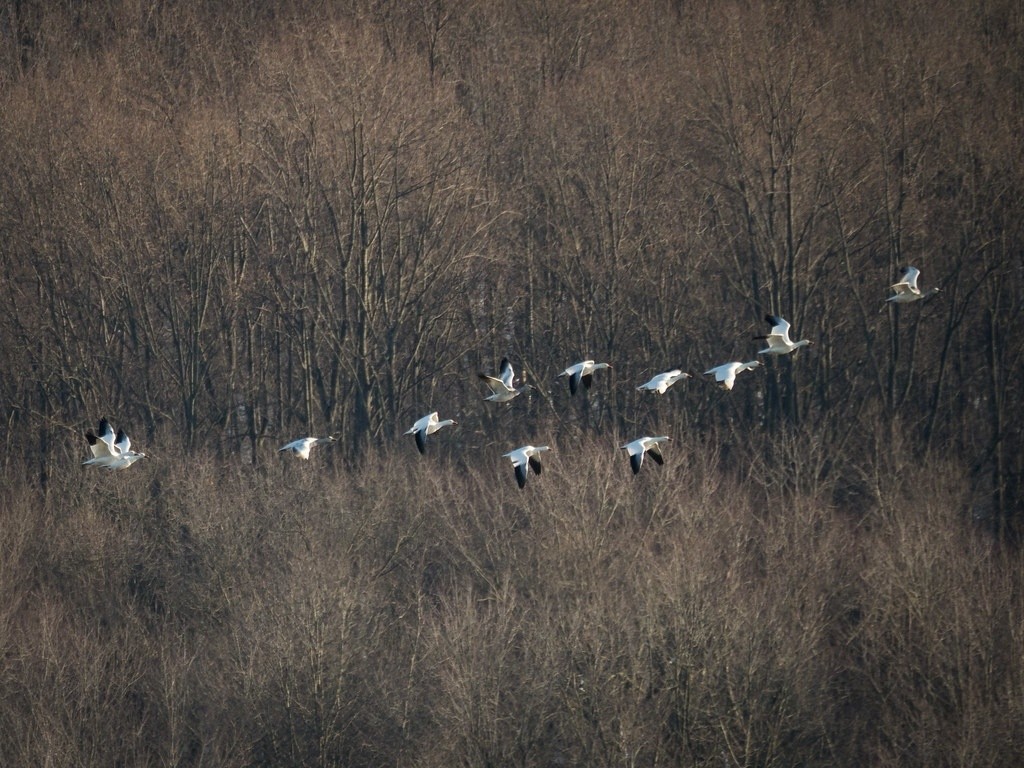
[501,445,552,489]
[81,416,150,473]
[636,369,692,394]
[702,361,764,391]
[401,411,458,455]
[885,266,945,303]
[749,313,815,355]
[619,435,673,475]
[557,360,613,396]
[476,356,536,403]
[276,436,338,460]
